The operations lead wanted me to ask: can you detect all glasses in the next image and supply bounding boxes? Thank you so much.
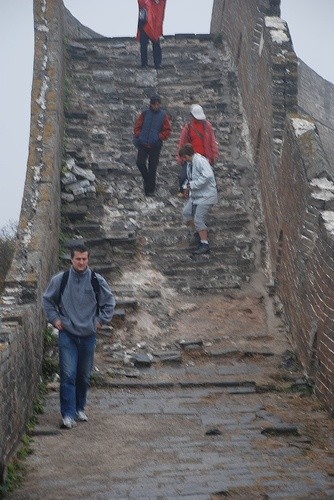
[180,151,190,157]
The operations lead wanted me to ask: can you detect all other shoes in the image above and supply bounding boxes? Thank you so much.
[156,63,162,69]
[193,242,209,255]
[60,416,73,429]
[147,192,155,197]
[143,65,149,70]
[75,410,88,422]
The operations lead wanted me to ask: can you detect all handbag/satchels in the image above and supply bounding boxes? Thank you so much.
[184,189,190,199]
[139,8,146,27]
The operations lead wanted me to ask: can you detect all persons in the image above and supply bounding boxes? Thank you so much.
[136,0,166,70]
[133,95,171,197]
[43,244,116,429]
[178,104,219,198]
[178,143,218,254]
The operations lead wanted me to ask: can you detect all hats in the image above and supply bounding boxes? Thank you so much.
[190,104,206,120]
